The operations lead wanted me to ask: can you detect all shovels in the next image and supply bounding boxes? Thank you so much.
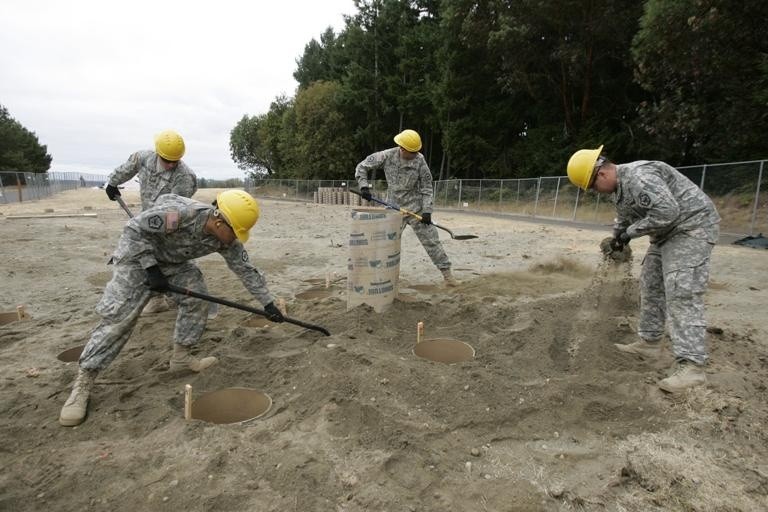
[115,194,219,320]
[349,188,479,240]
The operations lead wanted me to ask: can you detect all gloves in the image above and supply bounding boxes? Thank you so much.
[361,187,371,201]
[146,265,168,292]
[106,185,121,200]
[264,302,284,323]
[610,229,630,254]
[422,213,431,225]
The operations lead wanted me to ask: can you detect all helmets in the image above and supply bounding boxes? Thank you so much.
[217,191,259,243]
[567,145,604,191]
[155,131,185,161]
[394,129,422,152]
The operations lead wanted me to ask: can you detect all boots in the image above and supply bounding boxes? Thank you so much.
[170,343,218,372]
[659,358,707,394]
[59,377,94,426]
[442,268,466,286]
[615,339,664,361]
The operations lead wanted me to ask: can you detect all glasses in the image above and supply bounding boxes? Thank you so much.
[588,165,600,191]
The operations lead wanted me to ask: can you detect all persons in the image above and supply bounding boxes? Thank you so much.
[59,188,260,428]
[566,144,722,394]
[105,129,198,314]
[355,129,466,288]
